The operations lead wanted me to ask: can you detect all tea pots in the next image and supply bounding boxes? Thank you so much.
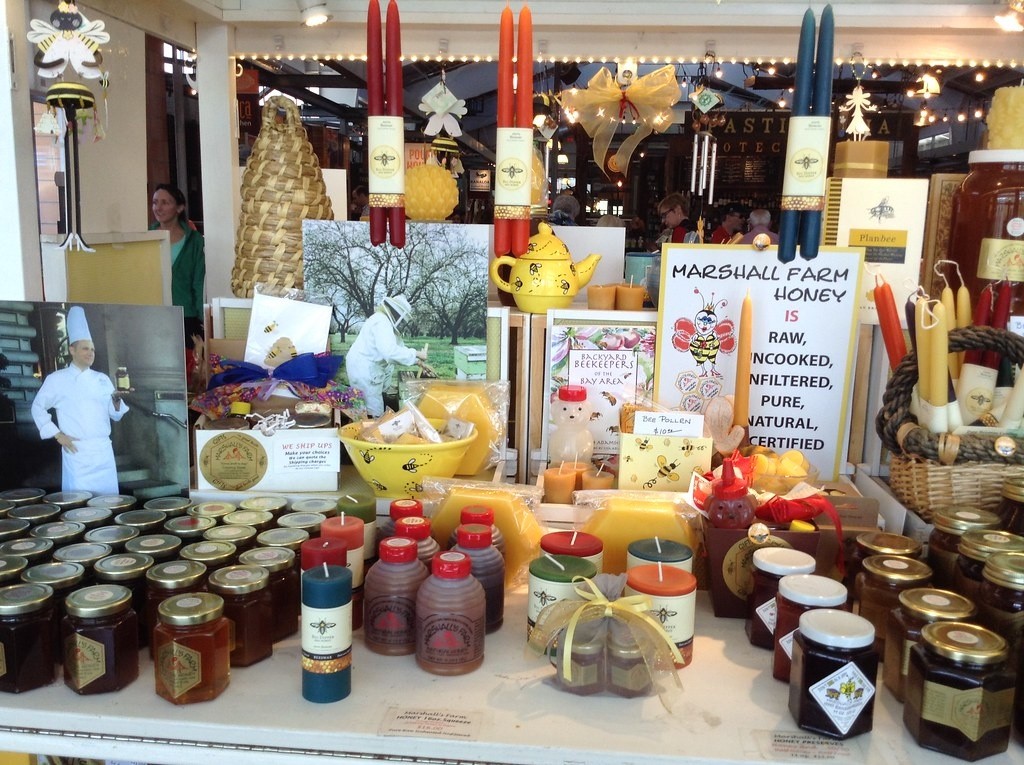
[490,222,603,314]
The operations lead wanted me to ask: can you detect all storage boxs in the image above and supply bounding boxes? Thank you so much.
[618,424,713,492]
[698,460,937,618]
[192,394,354,492]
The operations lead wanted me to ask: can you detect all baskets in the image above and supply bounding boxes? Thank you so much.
[889,450,1024,527]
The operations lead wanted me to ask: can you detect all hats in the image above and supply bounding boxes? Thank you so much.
[723,202,753,214]
[384,295,413,324]
[67,305,94,344]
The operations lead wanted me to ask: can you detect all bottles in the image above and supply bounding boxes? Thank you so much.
[375,499,504,563]
[560,627,652,698]
[416,551,486,676]
[448,523,504,634]
[364,536,430,656]
[704,458,754,529]
[394,515,440,574]
[549,385,593,467]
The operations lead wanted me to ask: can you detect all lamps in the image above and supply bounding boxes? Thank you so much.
[296,0,333,28]
[994,0,1024,32]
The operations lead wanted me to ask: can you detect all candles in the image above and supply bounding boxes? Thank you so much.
[405,152,459,224]
[301,493,377,704]
[544,451,613,504]
[367,0,404,248]
[873,258,1024,435]
[983,79,1024,151]
[588,274,645,311]
[835,129,888,180]
[494,0,534,260]
[778,0,835,264]
[526,529,697,669]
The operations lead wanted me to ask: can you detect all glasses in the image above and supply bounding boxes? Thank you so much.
[732,213,747,219]
[660,209,673,221]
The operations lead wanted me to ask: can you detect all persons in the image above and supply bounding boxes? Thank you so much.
[563,189,574,195]
[149,182,205,392]
[547,195,580,226]
[346,294,429,420]
[352,185,370,221]
[597,215,645,240]
[709,202,747,245]
[31,336,136,496]
[739,209,779,244]
[642,191,698,251]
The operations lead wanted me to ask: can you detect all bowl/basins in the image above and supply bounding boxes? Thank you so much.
[337,419,477,499]
[749,463,820,496]
